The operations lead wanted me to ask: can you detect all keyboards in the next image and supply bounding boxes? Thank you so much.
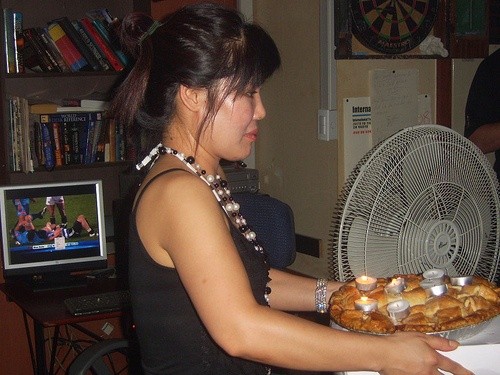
[63,290,128,316]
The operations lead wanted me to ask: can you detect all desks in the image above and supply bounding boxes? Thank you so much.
[0,269,129,375]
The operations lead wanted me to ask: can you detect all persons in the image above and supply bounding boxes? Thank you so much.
[107,2,475,375]
[463,48,500,194]
[10,195,98,245]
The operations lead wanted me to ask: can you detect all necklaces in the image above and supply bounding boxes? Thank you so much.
[136,142,272,307]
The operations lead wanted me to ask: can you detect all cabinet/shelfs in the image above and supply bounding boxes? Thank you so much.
[0,0,157,254]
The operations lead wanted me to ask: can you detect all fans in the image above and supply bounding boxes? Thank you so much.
[330,124,500,289]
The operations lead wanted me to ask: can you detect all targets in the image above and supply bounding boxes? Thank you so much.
[348,0,438,55]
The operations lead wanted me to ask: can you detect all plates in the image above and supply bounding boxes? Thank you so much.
[328,316,495,342]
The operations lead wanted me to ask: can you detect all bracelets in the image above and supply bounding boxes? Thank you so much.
[315,278,329,313]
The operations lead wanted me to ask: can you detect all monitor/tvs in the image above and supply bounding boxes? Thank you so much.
[0,180,107,292]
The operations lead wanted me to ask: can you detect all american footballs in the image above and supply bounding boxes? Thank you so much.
[50,224,57,230]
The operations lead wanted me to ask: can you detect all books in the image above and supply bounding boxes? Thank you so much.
[3,8,128,73]
[7,93,147,176]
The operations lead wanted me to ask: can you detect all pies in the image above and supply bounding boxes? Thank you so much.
[328,271,500,336]
